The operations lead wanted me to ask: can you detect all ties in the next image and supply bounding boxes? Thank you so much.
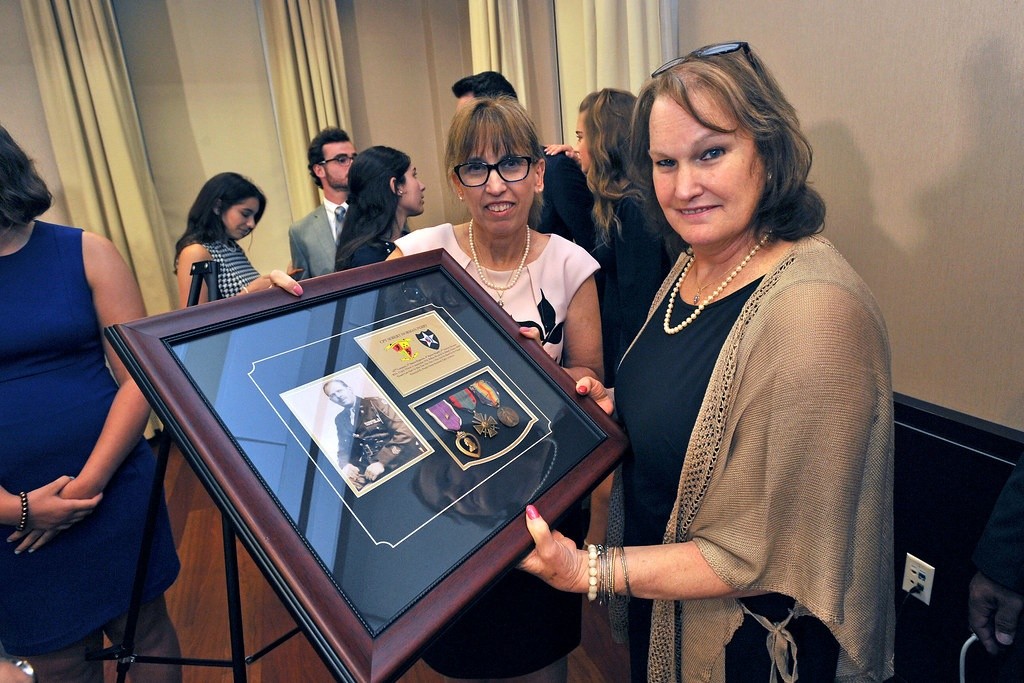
[334,205,346,244]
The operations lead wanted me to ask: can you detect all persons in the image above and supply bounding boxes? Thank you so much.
[452,70,623,388]
[173,172,271,308]
[384,94,603,683]
[520,41,895,683]
[323,379,427,490]
[0,125,182,683]
[412,413,599,528]
[334,146,425,272]
[544,87,672,346]
[968,452,1024,654]
[288,127,358,283]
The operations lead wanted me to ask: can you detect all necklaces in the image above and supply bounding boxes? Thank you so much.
[527,434,557,503]
[664,230,771,335]
[469,218,530,306]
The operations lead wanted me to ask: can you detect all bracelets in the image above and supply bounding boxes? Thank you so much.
[243,287,248,293]
[587,544,631,607]
[15,491,28,532]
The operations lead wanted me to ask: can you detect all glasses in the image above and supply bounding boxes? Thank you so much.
[652,41,769,92]
[453,156,539,187]
[318,154,358,167]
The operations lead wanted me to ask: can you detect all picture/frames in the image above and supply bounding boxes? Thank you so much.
[103,247,631,683]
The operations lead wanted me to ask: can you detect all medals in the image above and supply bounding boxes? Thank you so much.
[472,412,500,438]
[497,407,519,427]
[455,431,481,458]
